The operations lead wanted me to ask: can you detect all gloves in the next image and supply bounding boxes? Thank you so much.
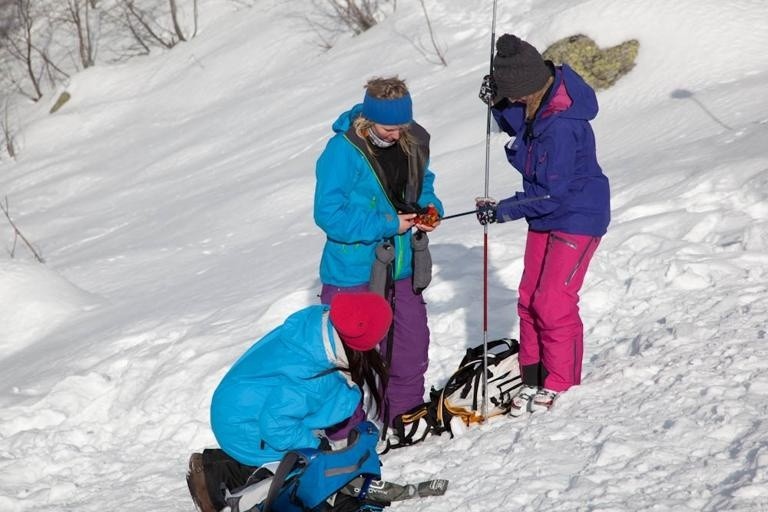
[475,200,497,225]
[369,243,396,299]
[412,233,433,294]
[478,76,497,107]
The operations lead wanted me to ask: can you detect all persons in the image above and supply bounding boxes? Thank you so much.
[185,289,395,512]
[312,72,444,429]
[478,32,611,418]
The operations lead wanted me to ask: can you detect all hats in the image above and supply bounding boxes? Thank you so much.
[329,292,393,352]
[494,34,550,97]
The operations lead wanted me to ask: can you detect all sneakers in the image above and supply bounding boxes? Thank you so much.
[510,385,542,417]
[186,452,217,512]
[531,388,564,413]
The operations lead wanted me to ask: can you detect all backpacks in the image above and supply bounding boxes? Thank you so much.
[262,419,390,512]
[430,338,525,439]
